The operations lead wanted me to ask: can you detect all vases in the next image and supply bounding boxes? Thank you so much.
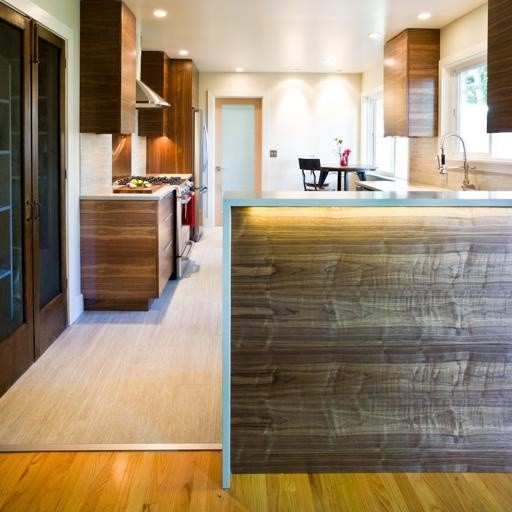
[338,153,348,166]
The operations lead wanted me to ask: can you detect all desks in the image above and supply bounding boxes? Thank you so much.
[316,165,377,191]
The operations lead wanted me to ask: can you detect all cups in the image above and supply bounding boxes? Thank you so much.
[341,160,346,166]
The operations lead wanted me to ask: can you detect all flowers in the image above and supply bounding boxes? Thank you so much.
[334,138,350,165]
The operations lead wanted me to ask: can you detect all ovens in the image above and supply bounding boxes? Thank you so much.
[175,193,193,280]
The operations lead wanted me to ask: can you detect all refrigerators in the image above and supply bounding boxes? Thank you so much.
[199,108,210,225]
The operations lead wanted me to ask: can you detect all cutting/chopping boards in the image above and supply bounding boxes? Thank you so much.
[113,183,164,194]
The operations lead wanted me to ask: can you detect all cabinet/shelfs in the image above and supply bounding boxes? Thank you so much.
[486,0,511,133]
[79,188,178,312]
[380,28,440,139]
[168,59,199,111]
[79,1,138,135]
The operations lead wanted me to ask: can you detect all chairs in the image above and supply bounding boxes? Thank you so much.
[298,157,336,193]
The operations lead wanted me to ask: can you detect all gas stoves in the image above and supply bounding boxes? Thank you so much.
[118,175,193,198]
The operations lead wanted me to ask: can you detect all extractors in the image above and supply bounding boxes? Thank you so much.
[135,30,172,110]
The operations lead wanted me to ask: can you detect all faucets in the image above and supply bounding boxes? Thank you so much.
[437,132,468,183]
